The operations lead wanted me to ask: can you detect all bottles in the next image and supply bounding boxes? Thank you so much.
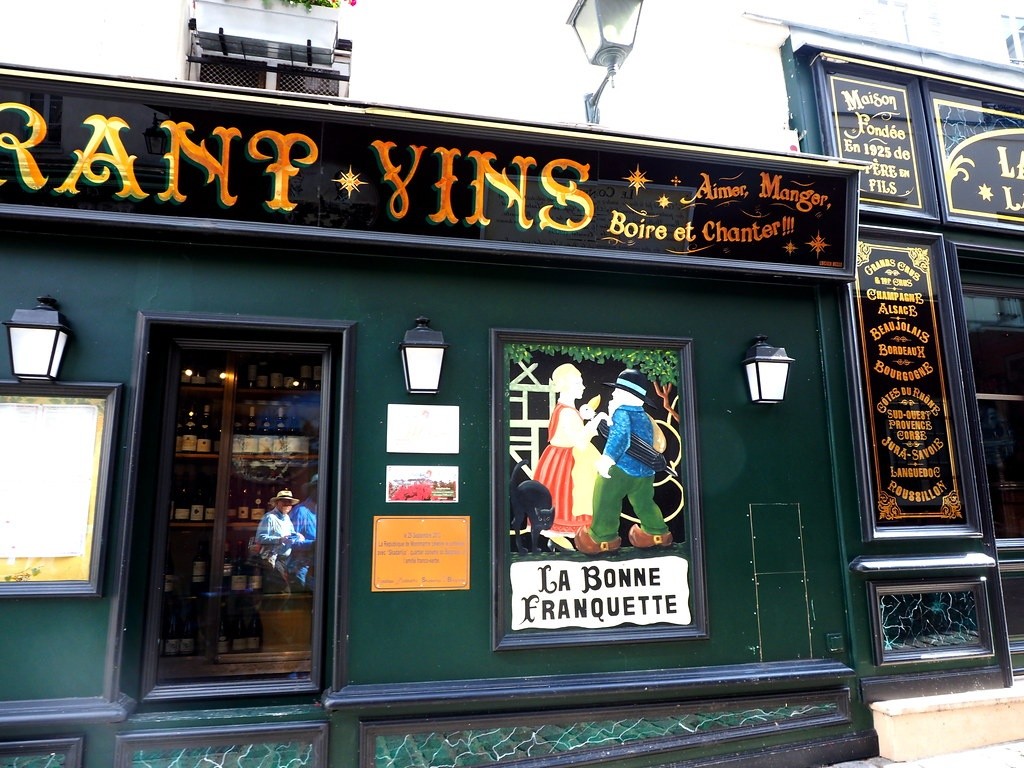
[213,417,222,454]
[175,472,190,522]
[232,406,245,453]
[231,600,247,653]
[196,405,212,453]
[248,601,263,653]
[232,541,247,593]
[181,350,223,386]
[238,354,270,388]
[164,599,196,656]
[192,541,207,593]
[222,543,232,592]
[176,422,183,452]
[183,403,199,453]
[247,545,262,593]
[162,558,176,602]
[244,407,258,454]
[168,473,177,522]
[216,602,230,654]
[300,355,321,389]
[259,406,304,454]
[190,473,217,523]
[226,486,291,522]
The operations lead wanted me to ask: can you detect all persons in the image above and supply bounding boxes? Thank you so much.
[255,491,304,593]
[287,473,318,593]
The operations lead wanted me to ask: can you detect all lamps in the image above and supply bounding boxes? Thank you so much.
[400,314,449,392]
[3,292,75,384]
[564,1,644,122]
[745,335,794,403]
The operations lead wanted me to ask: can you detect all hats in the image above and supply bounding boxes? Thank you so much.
[270,491,300,507]
[301,473,318,493]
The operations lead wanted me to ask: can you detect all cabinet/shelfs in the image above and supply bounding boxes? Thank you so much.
[160,339,329,679]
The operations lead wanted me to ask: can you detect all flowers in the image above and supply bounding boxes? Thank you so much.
[263,0,358,12]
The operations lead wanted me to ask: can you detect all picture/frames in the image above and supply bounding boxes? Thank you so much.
[485,327,712,652]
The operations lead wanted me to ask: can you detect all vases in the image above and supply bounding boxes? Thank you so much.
[193,0,349,65]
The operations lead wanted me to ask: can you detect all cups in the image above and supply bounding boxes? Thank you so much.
[271,373,283,388]
[284,377,294,389]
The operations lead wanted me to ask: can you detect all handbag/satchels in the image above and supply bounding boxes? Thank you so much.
[244,543,283,569]
